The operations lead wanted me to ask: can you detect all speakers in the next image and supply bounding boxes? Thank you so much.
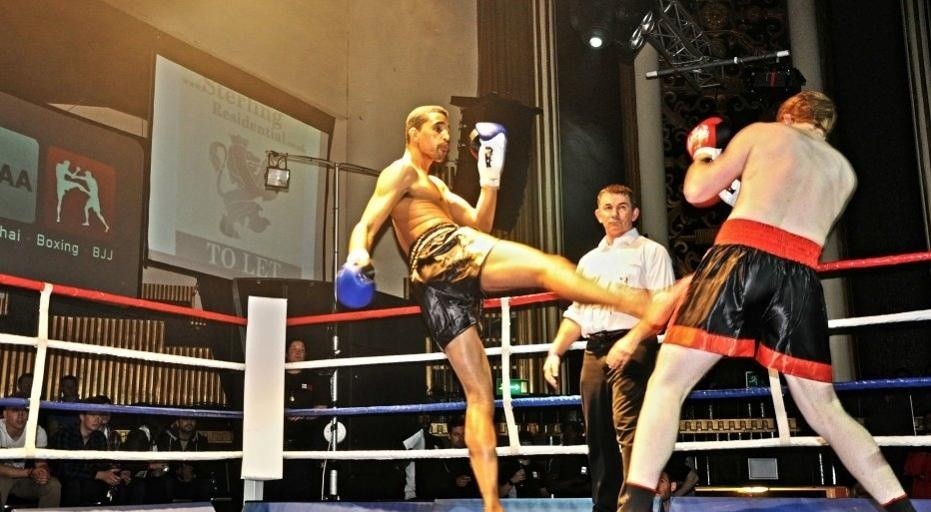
[451,96,543,233]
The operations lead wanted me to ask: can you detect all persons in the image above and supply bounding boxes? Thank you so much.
[542,184,675,512]
[903,448,931,498]
[614,90,913,512]
[336,104,693,512]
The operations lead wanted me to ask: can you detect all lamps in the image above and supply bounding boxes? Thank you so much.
[579,0,649,58]
[265,151,292,189]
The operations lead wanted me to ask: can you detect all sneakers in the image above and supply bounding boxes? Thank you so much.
[673,470,698,496]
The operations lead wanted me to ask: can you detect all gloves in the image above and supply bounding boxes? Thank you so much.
[687,117,722,161]
[335,250,376,310]
[718,179,740,207]
[466,121,507,189]
[543,353,562,389]
